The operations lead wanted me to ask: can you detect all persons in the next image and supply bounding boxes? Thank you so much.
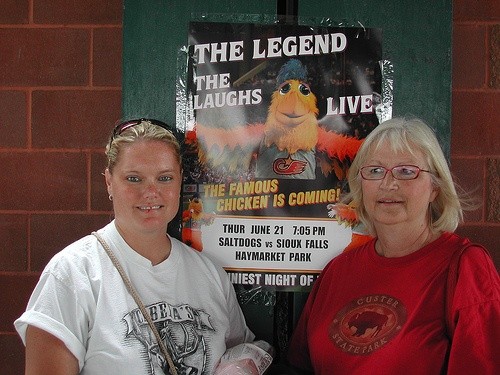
[279,113,500,375]
[13,118,257,375]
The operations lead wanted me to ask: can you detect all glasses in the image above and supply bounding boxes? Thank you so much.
[110,118,179,148]
[360,165,431,180]
